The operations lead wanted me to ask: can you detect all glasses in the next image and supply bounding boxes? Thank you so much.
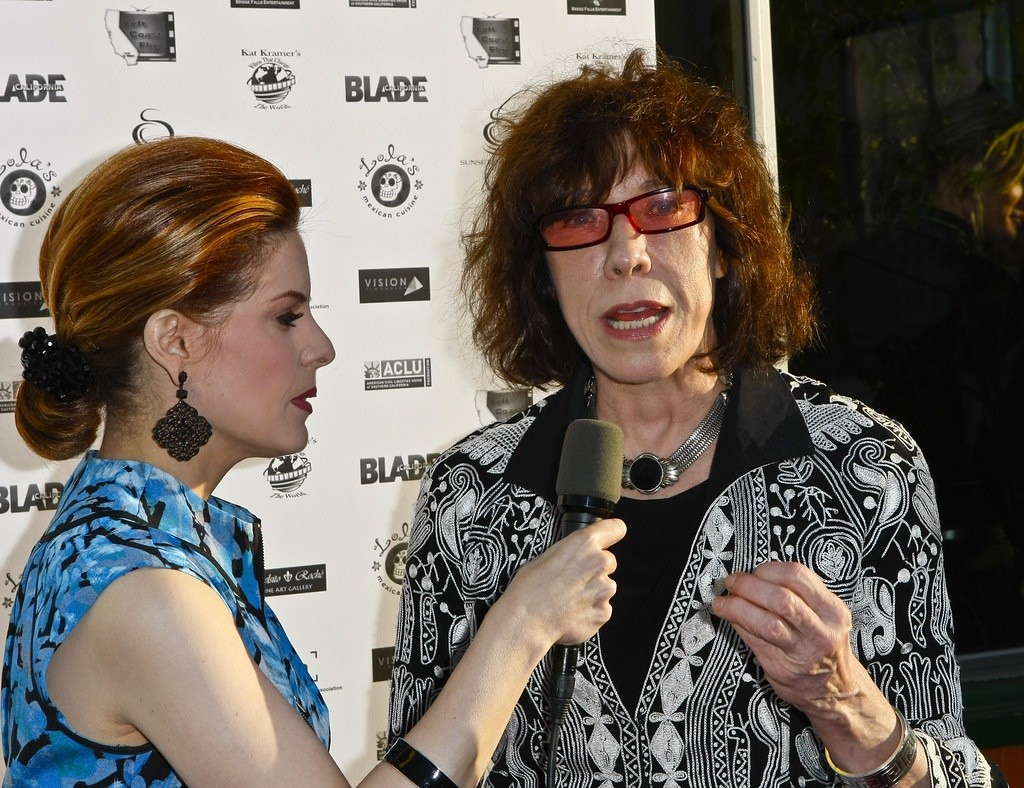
[529,185,713,251]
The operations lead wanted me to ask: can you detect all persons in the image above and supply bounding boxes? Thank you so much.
[791,98,1024,656]
[387,47,1011,788]
[0,136,628,788]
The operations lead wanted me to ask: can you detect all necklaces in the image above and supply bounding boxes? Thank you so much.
[584,371,733,494]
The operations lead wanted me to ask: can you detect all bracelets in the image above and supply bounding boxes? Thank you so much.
[824,707,917,788]
[382,737,460,787]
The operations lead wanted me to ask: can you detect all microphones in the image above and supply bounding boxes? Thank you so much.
[549,419,624,724]
